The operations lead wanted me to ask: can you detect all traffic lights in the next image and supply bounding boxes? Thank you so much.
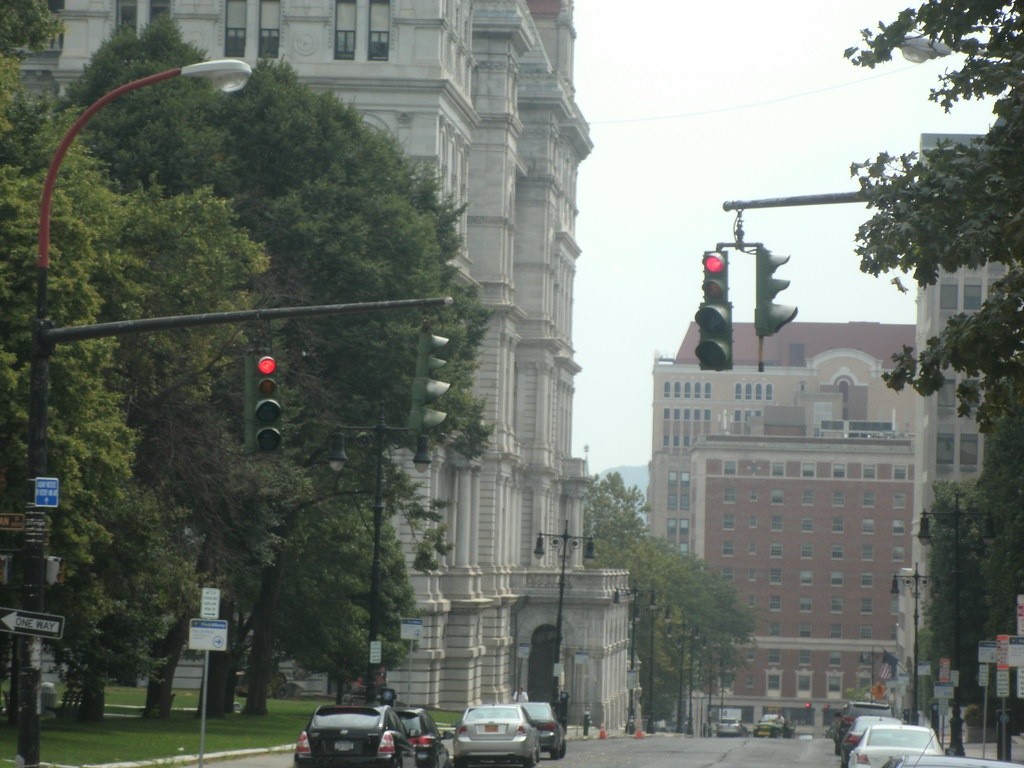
[806,703,810,711]
[243,343,283,454]
[702,249,730,305]
[406,319,451,436]
[693,302,735,373]
[755,245,798,341]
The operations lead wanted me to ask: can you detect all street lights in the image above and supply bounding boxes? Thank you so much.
[612,578,657,727]
[8,58,252,768]
[917,487,1000,759]
[888,562,940,724]
[326,399,433,707]
[532,519,598,721]
[633,600,725,734]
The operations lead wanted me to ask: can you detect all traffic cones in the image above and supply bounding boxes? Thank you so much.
[596,720,609,740]
[633,719,649,739]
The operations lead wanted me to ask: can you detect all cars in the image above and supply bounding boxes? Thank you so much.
[520,702,567,761]
[753,712,795,739]
[837,717,904,768]
[824,701,893,755]
[391,707,455,768]
[879,754,1023,768]
[715,717,744,737]
[847,721,945,768]
[451,702,543,768]
[292,704,420,768]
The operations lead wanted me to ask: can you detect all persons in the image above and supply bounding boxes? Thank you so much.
[513,687,528,704]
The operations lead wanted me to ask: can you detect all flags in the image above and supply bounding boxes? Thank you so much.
[879,651,898,680]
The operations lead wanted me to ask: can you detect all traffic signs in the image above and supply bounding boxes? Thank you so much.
[0,607,65,641]
